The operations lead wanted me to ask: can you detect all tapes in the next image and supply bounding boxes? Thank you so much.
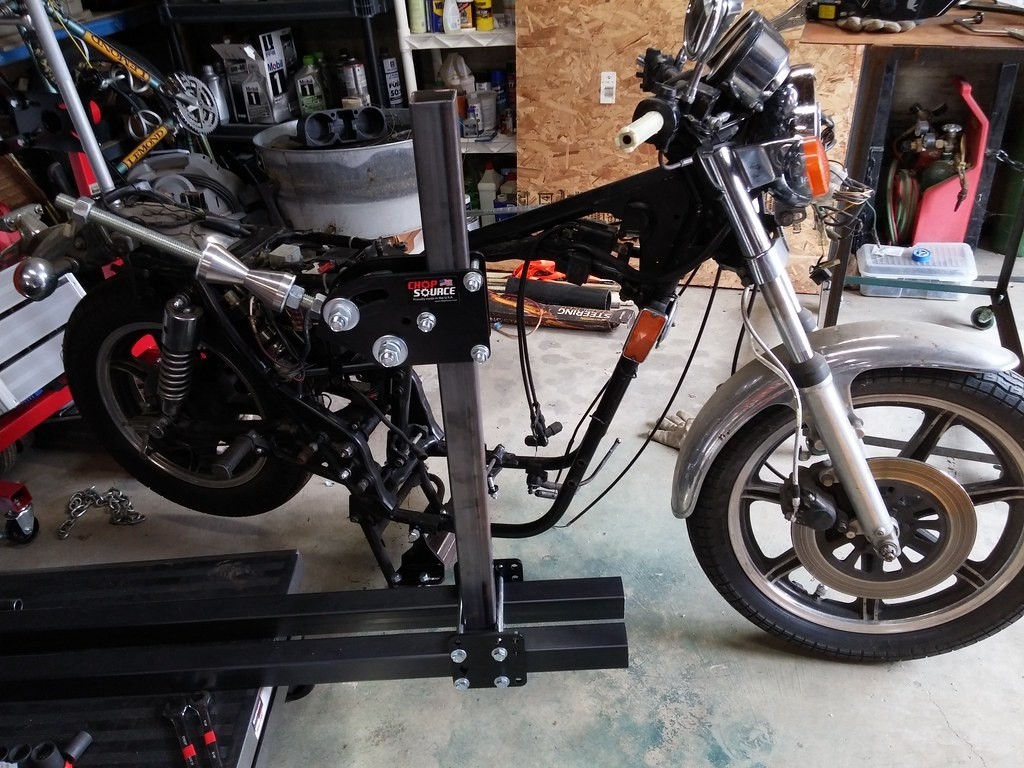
[911,248,932,262]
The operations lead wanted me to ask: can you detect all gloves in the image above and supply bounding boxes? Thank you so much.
[649,410,695,448]
[835,16,924,33]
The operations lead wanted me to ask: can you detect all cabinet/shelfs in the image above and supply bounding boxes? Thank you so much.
[157,0,424,153]
[394,0,517,154]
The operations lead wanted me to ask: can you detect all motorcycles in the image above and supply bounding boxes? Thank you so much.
[0,1,1023,668]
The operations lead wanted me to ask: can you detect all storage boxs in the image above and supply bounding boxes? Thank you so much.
[210,27,299,123]
[856,243,979,302]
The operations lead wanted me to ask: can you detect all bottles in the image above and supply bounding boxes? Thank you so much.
[474,0,493,31]
[443,0,461,33]
[214,61,234,113]
[408,0,427,33]
[201,64,230,125]
[377,47,403,109]
[464,162,517,231]
[242,63,274,124]
[456,59,516,138]
[295,51,371,113]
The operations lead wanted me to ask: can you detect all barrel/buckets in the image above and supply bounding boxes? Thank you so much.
[440,52,476,92]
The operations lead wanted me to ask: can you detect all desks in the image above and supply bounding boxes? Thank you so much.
[798,5,1024,256]
[0,0,157,92]
[0,549,315,768]
